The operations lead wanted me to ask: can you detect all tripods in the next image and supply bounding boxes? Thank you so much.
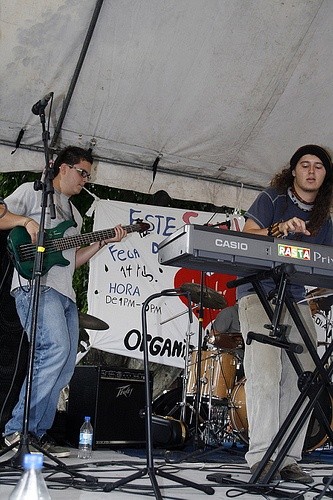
[0,114,98,483]
[103,291,226,500]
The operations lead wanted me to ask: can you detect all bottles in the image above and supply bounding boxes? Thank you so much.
[8,454,51,500]
[78,416,94,459]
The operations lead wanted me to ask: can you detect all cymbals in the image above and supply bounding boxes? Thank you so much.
[181,283,227,309]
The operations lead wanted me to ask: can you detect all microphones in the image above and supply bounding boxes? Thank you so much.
[31,92,54,115]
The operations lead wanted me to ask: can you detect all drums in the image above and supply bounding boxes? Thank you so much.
[228,378,332,454]
[186,348,239,402]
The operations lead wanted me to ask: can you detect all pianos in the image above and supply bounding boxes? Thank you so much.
[157,224,333,500]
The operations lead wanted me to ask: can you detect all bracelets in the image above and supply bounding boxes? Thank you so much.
[99,240,108,249]
[268,221,283,235]
[24,218,34,226]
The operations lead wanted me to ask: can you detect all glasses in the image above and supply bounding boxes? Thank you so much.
[59,164,91,181]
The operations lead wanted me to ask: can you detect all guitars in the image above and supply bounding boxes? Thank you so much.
[7,218,150,280]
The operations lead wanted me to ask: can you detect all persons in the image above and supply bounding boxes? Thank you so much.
[0,145,127,458]
[236,144,333,483]
[209,304,240,337]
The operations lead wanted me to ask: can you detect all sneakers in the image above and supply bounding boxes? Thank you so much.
[250,460,281,483]
[6,431,71,457]
[281,463,315,483]
